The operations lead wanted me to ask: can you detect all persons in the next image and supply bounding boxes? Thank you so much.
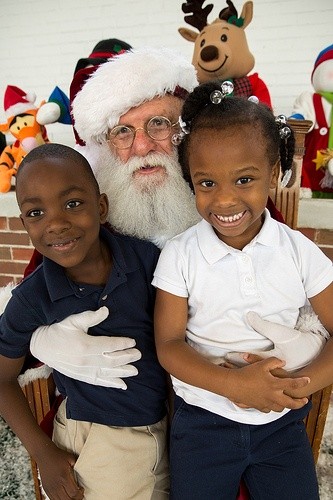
[0,38,333,500]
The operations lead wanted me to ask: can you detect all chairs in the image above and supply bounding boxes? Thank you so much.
[14,116,333,500]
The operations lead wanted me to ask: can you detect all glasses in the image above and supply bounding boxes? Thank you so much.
[106,115,179,150]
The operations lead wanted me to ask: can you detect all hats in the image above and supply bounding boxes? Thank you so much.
[4,85,37,120]
[69,38,200,147]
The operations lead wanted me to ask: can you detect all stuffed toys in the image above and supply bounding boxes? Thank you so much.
[178,0,271,109]
[0,85,60,193]
[311,45,333,175]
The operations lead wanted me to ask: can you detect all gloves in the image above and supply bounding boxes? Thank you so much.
[223,311,327,375]
[30,306,142,391]
